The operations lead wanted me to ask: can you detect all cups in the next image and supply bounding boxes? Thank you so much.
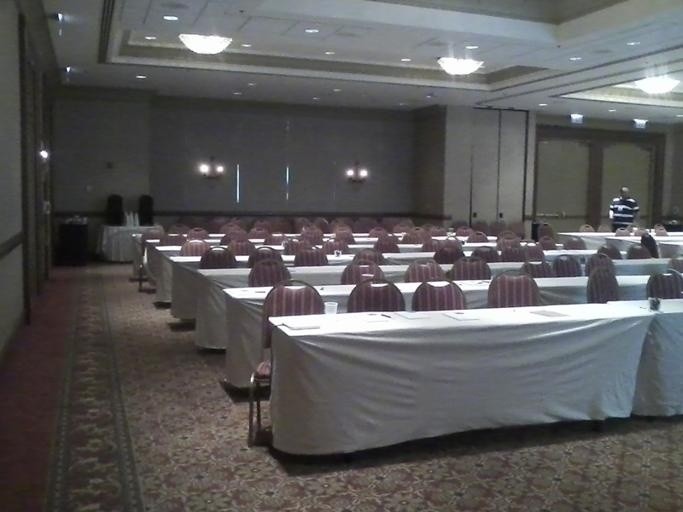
[323,302,338,316]
[334,251,341,257]
[649,298,660,310]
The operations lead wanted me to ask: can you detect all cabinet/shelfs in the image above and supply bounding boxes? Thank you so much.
[60,219,88,266]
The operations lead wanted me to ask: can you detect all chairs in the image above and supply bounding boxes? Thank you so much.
[131,218,683,440]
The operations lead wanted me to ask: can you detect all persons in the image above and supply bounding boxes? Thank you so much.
[608,186,640,233]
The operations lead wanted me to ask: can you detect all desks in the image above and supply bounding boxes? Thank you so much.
[265,297,682,454]
[95,223,164,265]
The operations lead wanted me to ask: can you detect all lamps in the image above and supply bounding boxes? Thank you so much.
[434,56,484,77]
[635,75,680,95]
[198,155,228,180]
[177,33,233,55]
[345,162,369,182]
